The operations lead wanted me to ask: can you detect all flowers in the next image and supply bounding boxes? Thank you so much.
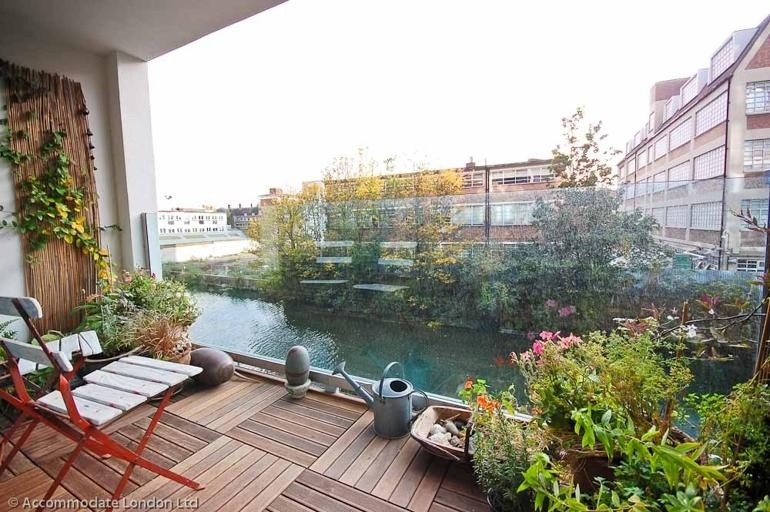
[457,377,518,427]
[509,331,583,430]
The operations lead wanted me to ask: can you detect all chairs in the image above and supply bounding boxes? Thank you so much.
[0,296,204,512]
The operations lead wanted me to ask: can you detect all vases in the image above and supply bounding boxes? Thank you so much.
[150,336,193,401]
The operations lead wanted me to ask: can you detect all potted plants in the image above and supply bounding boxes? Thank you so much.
[71,267,202,372]
[470,409,533,512]
